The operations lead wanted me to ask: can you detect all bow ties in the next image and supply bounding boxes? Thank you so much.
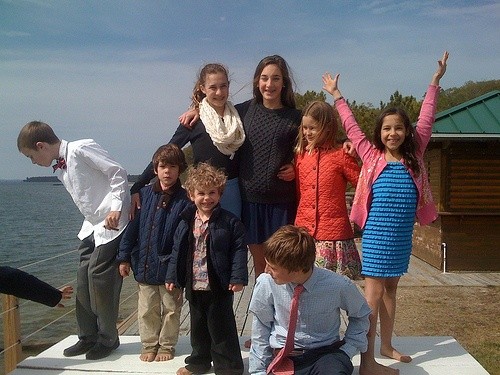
[52,157,67,174]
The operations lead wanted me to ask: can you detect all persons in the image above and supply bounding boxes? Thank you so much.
[17,120,131,360]
[321,51,450,375]
[294,100,365,281]
[0,266,74,308]
[130,64,296,220]
[249,224,373,375]
[178,54,358,348]
[116,143,198,362]
[176,162,248,375]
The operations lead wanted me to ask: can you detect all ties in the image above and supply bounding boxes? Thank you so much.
[266,286,306,375]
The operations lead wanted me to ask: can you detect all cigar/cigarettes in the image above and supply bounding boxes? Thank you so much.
[102,225,119,231]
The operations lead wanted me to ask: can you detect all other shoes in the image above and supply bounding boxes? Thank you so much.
[64,341,89,356]
[86,341,121,360]
